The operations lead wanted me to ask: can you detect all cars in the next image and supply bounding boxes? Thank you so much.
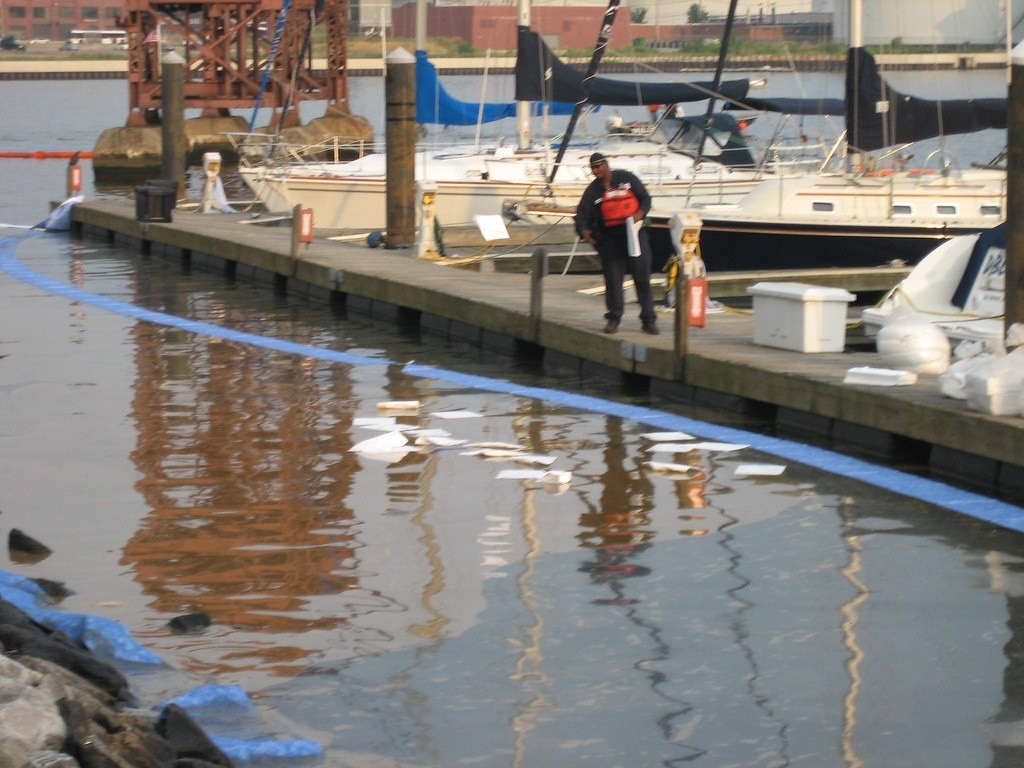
[60,40,79,51]
[0,36,27,51]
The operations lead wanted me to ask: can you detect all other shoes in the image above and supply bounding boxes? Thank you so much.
[603,315,620,333]
[644,319,659,334]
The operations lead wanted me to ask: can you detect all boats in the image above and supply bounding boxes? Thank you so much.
[239,0,1018,307]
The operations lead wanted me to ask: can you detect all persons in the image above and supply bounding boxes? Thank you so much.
[572,152,661,336]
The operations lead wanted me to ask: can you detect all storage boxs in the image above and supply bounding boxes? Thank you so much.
[746,281,857,354]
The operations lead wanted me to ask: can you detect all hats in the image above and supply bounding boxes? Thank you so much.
[590,153,607,168]
